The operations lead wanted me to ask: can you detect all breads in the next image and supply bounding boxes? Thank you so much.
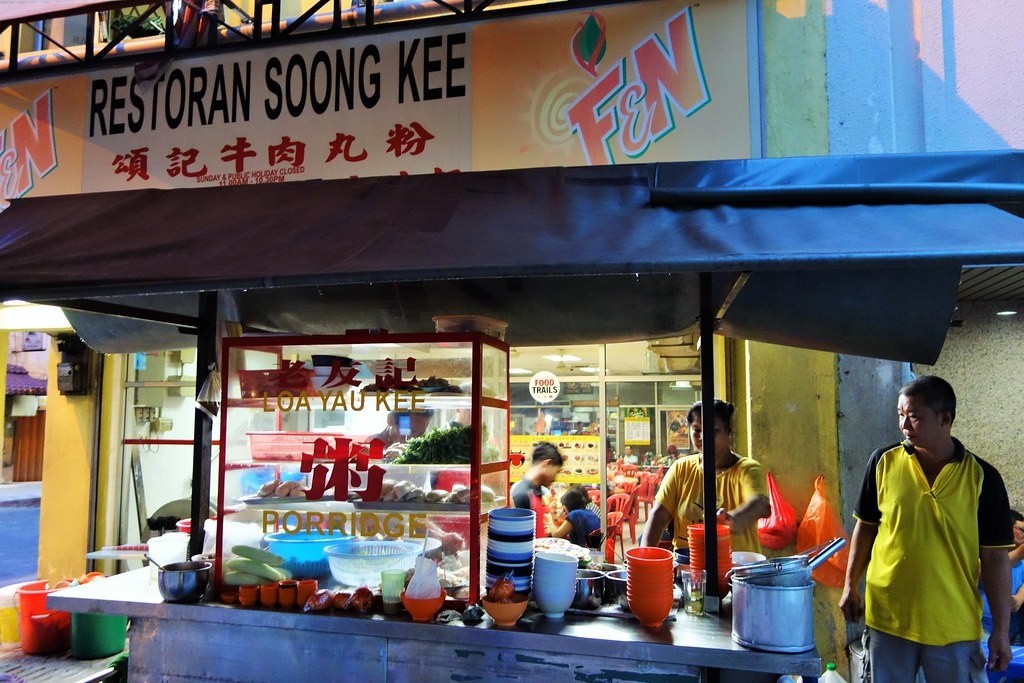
[259,480,309,496]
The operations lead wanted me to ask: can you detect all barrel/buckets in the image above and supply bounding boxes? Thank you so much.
[146,536,189,586]
[0,581,37,647]
[731,575,817,653]
[13,581,69,655]
[849,639,872,683]
[176,517,218,534]
[732,553,809,577]
[71,613,128,658]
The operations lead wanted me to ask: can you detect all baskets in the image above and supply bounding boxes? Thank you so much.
[263,529,356,576]
[325,540,417,589]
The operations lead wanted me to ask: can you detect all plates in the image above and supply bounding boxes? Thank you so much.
[550,441,599,475]
[358,382,497,398]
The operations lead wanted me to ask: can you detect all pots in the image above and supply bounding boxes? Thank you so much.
[735,537,846,585]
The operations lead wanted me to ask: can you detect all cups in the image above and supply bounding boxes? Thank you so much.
[680,568,707,617]
[381,569,405,614]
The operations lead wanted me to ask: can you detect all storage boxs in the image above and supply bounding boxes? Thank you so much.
[432,316,508,342]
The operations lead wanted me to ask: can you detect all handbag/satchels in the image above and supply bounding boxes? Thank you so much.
[797,475,852,588]
[756,471,797,550]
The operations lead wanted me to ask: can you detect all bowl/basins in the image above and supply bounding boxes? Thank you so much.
[309,354,353,397]
[52,572,106,590]
[400,590,447,621]
[480,506,768,632]
[263,529,357,577]
[157,553,318,607]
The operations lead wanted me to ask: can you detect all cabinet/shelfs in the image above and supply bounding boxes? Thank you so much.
[215,331,510,611]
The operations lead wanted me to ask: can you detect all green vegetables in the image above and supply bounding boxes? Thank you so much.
[391,421,499,469]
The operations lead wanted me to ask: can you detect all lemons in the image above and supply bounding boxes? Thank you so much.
[309,586,373,610]
[485,576,515,604]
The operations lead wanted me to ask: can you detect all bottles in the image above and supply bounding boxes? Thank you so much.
[817,663,847,683]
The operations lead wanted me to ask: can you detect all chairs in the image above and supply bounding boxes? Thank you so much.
[587,458,665,560]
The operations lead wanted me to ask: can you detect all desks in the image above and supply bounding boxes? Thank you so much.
[635,465,671,469]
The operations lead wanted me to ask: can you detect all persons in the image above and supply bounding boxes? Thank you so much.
[368,403,465,555]
[838,374,1024,683]
[426,382,498,546]
[656,445,687,465]
[560,418,639,465]
[510,443,601,549]
[640,400,772,551]
